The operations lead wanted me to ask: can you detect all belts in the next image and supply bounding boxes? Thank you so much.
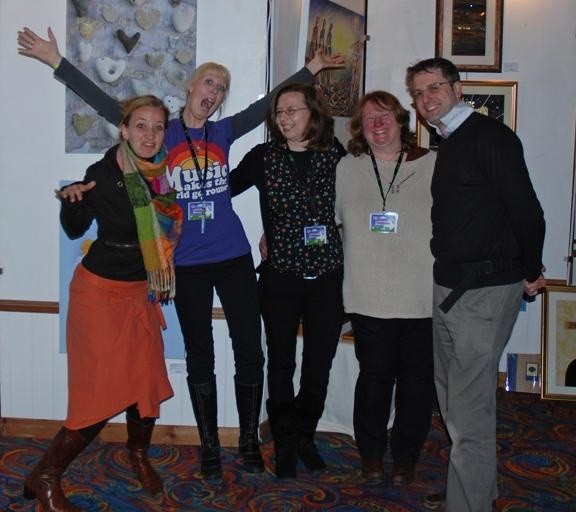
[277,271,338,281]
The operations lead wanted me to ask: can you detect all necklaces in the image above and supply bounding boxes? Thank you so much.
[184,123,204,151]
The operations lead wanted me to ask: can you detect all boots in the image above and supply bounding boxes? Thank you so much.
[182,377,226,482]
[268,394,328,478]
[120,415,165,495]
[20,426,97,512]
[232,375,265,474]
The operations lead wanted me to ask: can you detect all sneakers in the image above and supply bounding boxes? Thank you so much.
[359,455,417,488]
[424,489,496,506]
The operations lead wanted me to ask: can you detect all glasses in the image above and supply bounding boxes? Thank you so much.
[410,80,454,97]
[275,106,312,116]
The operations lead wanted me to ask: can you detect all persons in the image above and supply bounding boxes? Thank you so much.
[21,93,183,512]
[13,24,345,486]
[403,56,547,512]
[331,88,439,488]
[227,81,349,481]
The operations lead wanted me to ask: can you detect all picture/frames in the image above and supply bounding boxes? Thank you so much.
[539,281,575,404]
[433,0,506,74]
[412,79,520,154]
